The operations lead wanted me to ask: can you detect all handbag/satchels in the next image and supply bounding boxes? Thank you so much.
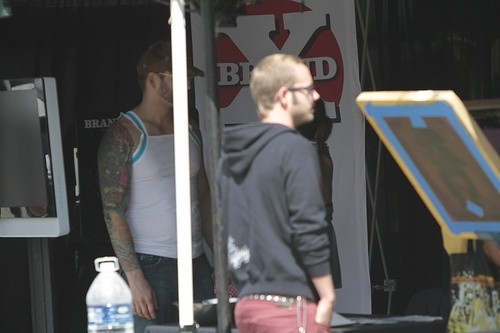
[444,239,500,333]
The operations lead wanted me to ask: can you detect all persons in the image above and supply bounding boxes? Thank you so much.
[96,41,215,333]
[215,52,337,333]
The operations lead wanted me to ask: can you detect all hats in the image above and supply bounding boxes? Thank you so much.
[137,39,205,78]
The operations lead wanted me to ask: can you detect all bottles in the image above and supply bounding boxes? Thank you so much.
[86,256,134,333]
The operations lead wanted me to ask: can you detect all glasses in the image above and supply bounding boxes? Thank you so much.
[287,83,315,94]
